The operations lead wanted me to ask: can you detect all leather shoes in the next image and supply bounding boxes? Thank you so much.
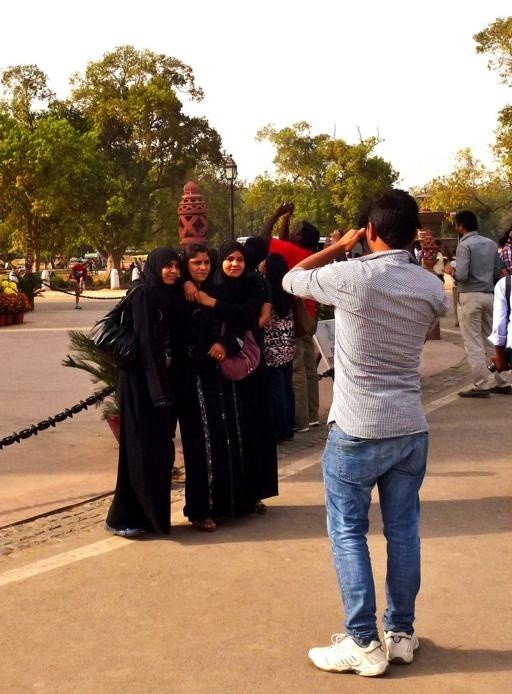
[490,386,511,395]
[457,388,489,397]
[104,523,146,537]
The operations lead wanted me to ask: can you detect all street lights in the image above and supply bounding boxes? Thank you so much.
[221,153,239,243]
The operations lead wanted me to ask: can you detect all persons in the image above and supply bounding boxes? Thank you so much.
[486,273,511,375]
[279,190,446,678]
[450,260,459,326]
[433,238,445,283]
[105,203,317,535]
[68,257,87,307]
[441,210,511,399]
[498,226,511,273]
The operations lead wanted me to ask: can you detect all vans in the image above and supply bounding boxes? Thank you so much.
[234,235,331,253]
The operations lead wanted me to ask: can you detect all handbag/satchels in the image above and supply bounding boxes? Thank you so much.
[218,329,260,381]
[264,312,296,366]
[87,304,140,364]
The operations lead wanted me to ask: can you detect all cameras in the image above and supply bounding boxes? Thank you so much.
[487,347,512,373]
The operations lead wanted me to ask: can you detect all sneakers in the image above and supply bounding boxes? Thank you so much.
[256,500,267,514]
[191,516,216,531]
[293,420,319,432]
[308,637,391,677]
[383,630,419,664]
